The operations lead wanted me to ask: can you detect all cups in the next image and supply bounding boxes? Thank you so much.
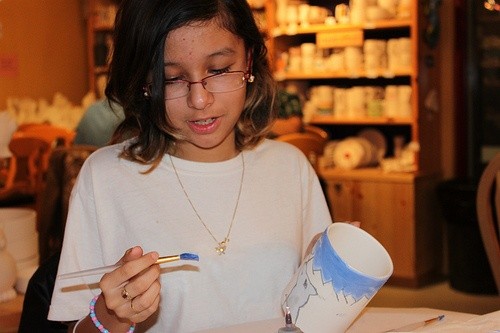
[281,222,394,333]
[274,0,409,31]
[288,37,410,71]
[307,84,411,118]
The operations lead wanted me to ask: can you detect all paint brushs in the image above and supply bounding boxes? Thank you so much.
[56,252,201,281]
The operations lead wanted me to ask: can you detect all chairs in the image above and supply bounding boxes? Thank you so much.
[0,120,98,232]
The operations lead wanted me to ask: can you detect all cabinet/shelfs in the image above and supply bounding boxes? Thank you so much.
[85,0,440,287]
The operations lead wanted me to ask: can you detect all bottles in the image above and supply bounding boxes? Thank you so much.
[278,306,303,333]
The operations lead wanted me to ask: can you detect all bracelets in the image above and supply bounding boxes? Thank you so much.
[90,295,136,333]
[73,313,88,333]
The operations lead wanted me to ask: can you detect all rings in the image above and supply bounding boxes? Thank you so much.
[122,287,132,300]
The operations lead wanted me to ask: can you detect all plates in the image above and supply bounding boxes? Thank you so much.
[331,129,386,170]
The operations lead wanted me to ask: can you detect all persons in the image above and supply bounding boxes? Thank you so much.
[54,93,138,237]
[49,0,332,333]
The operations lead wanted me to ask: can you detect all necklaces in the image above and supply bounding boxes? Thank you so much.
[168,144,244,254]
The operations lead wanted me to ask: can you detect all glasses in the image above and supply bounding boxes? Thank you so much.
[162,51,256,100]
